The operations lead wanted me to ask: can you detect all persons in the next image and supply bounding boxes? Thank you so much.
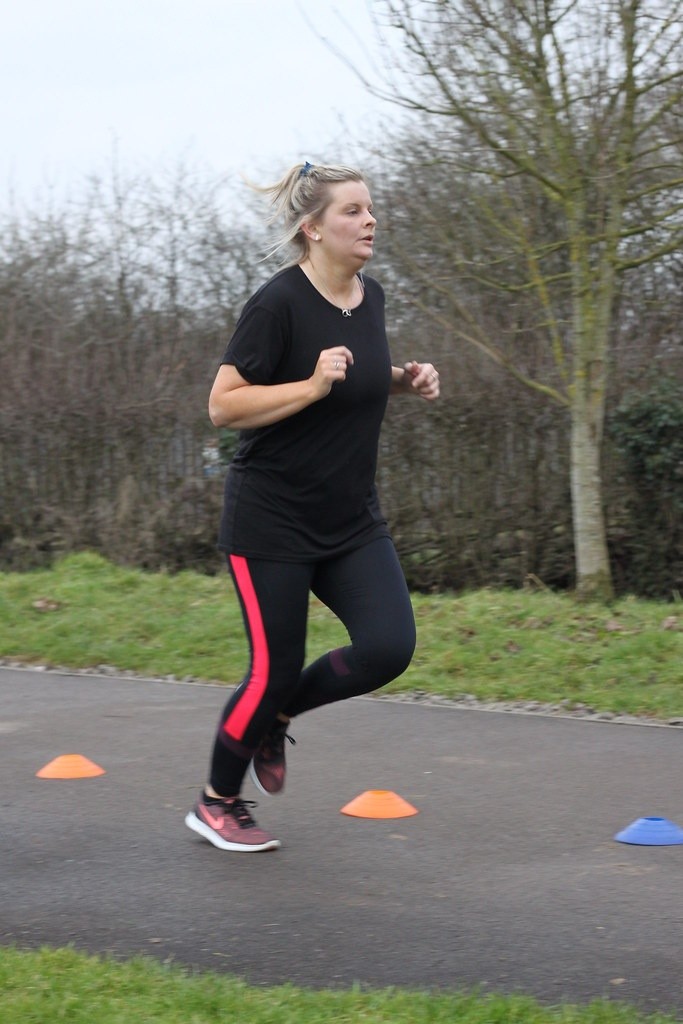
[185,162,441,850]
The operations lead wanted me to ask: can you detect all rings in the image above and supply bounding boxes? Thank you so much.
[335,362,340,370]
[431,374,436,382]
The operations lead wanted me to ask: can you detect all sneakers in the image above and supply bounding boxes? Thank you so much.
[184,790,280,852]
[249,716,290,796]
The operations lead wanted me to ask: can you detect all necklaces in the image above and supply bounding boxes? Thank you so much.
[307,256,357,317]
